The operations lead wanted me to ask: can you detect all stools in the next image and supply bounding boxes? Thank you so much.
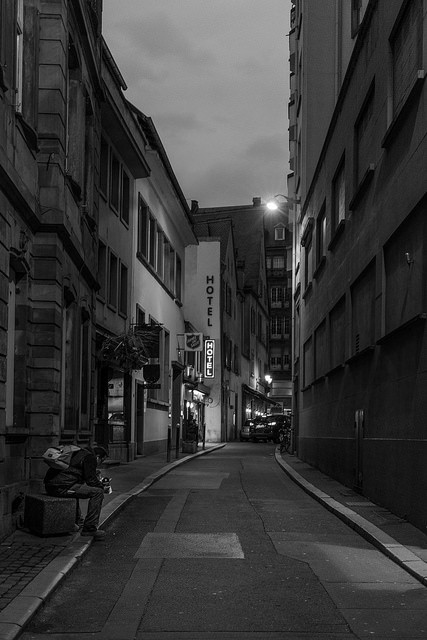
[24,493,76,537]
[183,441,197,454]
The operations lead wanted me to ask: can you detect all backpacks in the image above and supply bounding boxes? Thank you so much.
[42,444,83,470]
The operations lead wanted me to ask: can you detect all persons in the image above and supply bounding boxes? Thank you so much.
[272,417,284,444]
[43,445,111,538]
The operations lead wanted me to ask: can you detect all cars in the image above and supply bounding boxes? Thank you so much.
[239,419,253,442]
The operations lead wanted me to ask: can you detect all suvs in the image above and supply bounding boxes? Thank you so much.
[248,413,290,444]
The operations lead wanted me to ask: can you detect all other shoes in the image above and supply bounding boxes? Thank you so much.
[81,529,106,538]
[78,520,84,525]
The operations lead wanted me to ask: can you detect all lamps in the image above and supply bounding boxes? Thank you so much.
[266,193,292,208]
